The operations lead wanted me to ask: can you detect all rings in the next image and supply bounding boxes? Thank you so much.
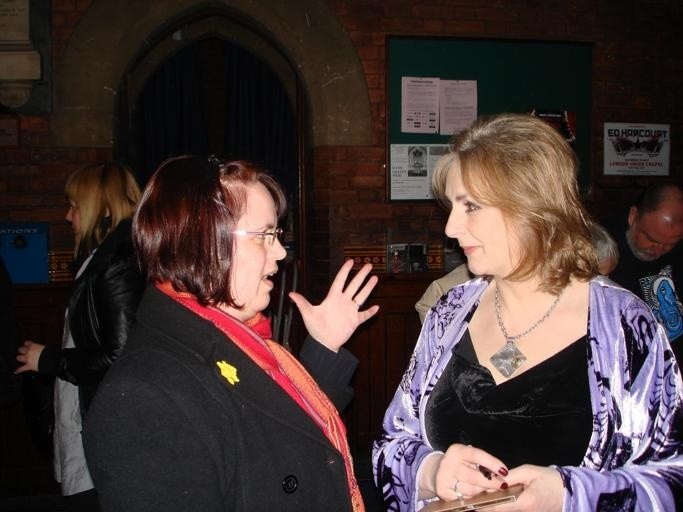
[451,480,459,489]
[351,296,363,307]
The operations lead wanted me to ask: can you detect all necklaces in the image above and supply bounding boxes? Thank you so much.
[492,280,562,380]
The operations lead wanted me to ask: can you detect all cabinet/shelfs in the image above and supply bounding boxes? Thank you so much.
[328,269,452,483]
[0,282,74,498]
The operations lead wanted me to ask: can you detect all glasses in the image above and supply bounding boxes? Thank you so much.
[225,226,285,249]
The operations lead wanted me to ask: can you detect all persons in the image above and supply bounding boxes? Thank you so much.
[595,179,682,368]
[11,161,153,509]
[413,214,620,330]
[368,110,683,511]
[75,155,383,512]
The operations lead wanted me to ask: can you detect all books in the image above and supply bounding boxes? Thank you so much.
[404,243,427,274]
[385,243,407,275]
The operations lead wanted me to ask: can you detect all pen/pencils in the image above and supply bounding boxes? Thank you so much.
[456,428,491,480]
